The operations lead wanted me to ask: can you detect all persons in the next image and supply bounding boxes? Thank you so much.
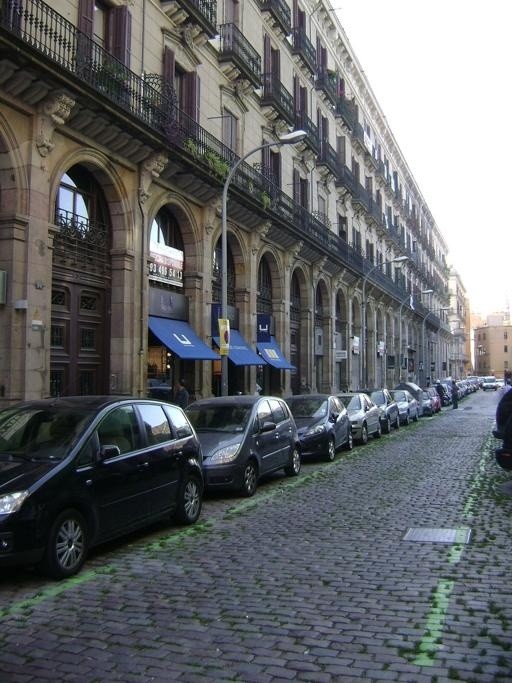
[175,379,188,409]
[450,381,460,408]
[255,382,262,395]
[436,379,446,406]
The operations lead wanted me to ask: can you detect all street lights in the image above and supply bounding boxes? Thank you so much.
[398,289,434,381]
[422,305,477,388]
[361,254,410,391]
[218,129,311,396]
